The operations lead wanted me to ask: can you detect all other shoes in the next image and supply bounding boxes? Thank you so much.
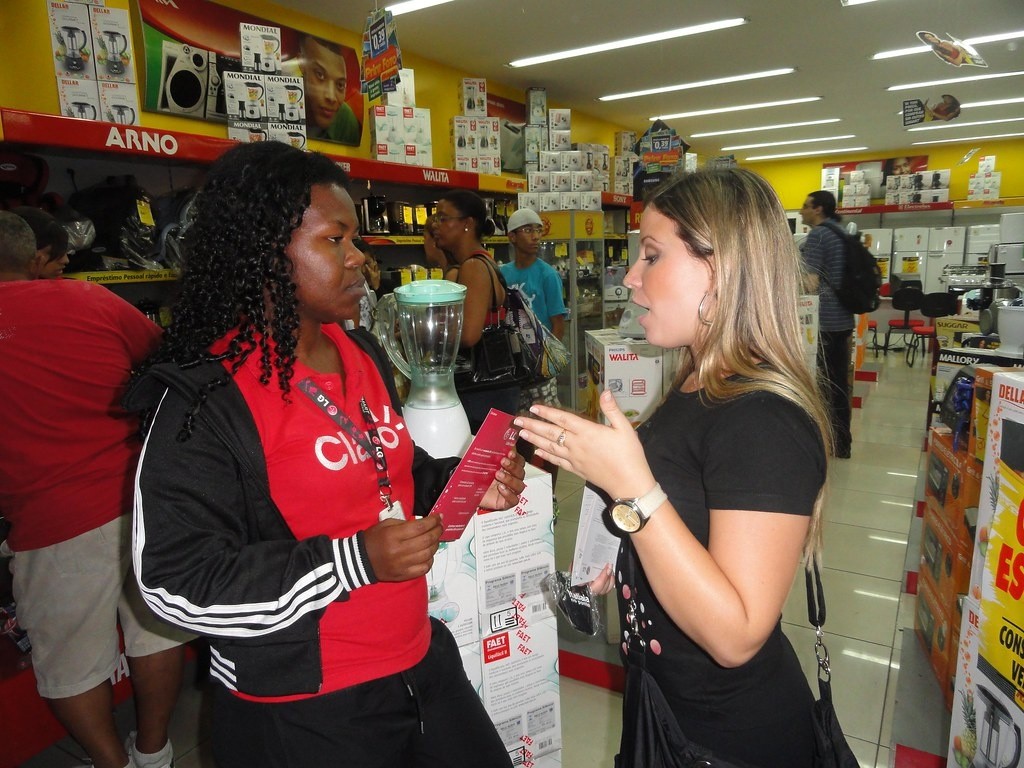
[833,450,850,458]
[124,732,175,768]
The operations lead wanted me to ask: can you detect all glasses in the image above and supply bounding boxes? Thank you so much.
[514,228,544,236]
[432,215,464,225]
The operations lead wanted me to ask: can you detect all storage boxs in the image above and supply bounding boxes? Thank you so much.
[222,21,309,152]
[884,169,949,205]
[425,292,818,768]
[841,170,872,208]
[367,70,433,168]
[912,347,1024,768]
[966,156,1001,201]
[518,87,636,212]
[447,77,502,178]
[685,153,698,174]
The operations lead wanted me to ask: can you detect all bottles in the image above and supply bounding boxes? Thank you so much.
[240,101,245,120]
[278,103,285,121]
[254,52,259,73]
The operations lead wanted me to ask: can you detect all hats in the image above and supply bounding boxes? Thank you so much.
[507,208,543,233]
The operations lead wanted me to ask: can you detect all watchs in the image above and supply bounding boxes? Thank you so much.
[610,484,667,533]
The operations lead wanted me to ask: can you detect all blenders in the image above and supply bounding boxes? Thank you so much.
[618,229,648,339]
[288,132,305,149]
[247,128,268,142]
[243,82,264,122]
[283,84,303,123]
[975,684,1021,768]
[372,279,474,459]
[260,34,279,73]
[110,104,136,125]
[61,25,87,72]
[387,112,426,166]
[457,86,490,174]
[587,152,609,191]
[102,31,128,75]
[425,543,464,623]
[72,102,96,120]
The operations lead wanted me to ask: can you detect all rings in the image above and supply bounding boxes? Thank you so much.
[558,429,566,447]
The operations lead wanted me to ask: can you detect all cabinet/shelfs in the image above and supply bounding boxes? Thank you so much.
[0,107,636,768]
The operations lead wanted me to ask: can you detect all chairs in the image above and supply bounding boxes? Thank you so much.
[865,288,959,368]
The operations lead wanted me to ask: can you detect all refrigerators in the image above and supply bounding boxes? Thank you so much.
[894,227,930,293]
[859,229,893,285]
[925,226,966,293]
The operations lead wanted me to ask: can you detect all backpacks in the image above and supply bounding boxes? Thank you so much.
[817,223,880,314]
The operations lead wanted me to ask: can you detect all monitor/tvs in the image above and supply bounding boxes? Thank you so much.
[889,272,922,297]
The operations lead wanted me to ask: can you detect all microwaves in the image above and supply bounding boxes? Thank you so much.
[985,212,1024,273]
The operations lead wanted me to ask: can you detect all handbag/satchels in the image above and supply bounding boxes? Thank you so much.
[453,254,571,393]
[614,648,861,767]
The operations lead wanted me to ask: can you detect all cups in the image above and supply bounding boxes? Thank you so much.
[996,304,1024,357]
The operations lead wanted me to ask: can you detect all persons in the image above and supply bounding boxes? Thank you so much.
[498,209,568,524]
[123,138,527,768]
[295,36,360,145]
[1,206,200,768]
[918,32,965,66]
[794,191,855,460]
[424,190,511,451]
[884,158,911,175]
[513,168,842,768]
[931,94,960,123]
